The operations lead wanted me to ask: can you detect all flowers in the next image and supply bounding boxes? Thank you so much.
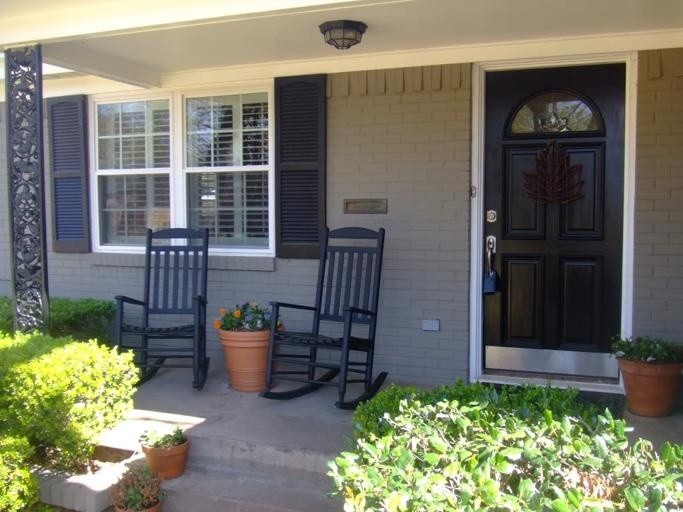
[611,334,683,363]
[213,300,281,331]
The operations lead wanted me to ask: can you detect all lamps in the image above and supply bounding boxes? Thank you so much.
[318,20,368,49]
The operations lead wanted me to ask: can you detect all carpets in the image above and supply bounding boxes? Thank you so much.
[481,382,624,421]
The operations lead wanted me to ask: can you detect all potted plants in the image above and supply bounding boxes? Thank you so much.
[108,466,168,512]
[142,427,192,480]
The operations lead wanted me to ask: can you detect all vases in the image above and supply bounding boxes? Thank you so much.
[217,324,284,394]
[616,358,683,417]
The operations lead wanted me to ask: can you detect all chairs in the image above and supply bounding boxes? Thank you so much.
[114,227,211,389]
[258,227,389,406]
